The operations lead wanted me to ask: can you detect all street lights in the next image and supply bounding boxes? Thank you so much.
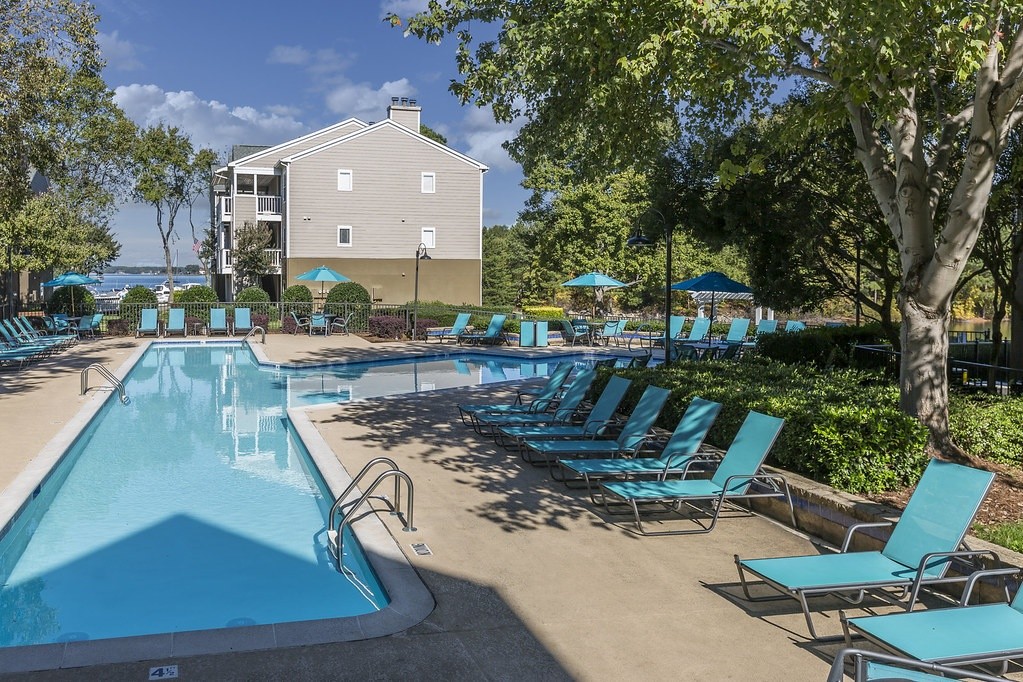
[628,207,672,369]
[413,242,432,342]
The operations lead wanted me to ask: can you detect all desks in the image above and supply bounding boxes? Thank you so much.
[307,314,336,336]
[192,323,205,337]
[576,322,605,347]
[65,317,81,320]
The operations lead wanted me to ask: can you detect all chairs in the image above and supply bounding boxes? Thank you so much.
[519,320,550,347]
[561,319,629,348]
[290,311,353,338]
[640,314,845,350]
[163,308,188,338]
[136,309,160,339]
[424,313,510,348]
[537,362,547,375]
[0,313,104,376]
[456,343,800,536]
[453,358,471,375]
[485,360,507,381]
[520,363,532,375]
[733,456,1023,682]
[232,308,255,337]
[207,308,229,337]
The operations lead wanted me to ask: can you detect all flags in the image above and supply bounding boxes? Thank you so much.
[193,238,202,252]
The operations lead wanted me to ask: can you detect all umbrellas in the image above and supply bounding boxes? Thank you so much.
[671,270,751,346]
[42,272,100,316]
[295,265,351,311]
[563,272,627,319]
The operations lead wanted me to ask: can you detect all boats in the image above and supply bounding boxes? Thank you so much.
[82,248,202,314]
